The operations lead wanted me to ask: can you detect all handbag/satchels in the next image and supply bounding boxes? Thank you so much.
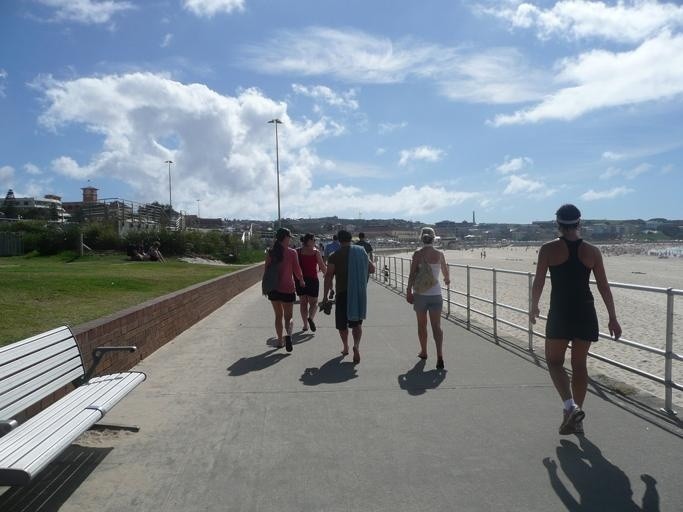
[413,264,438,293]
[262,263,278,294]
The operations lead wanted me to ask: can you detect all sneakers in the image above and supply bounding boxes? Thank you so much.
[317,290,335,314]
[302,318,316,332]
[437,359,444,368]
[417,351,427,358]
[274,340,284,347]
[285,335,294,352]
[558,403,585,434]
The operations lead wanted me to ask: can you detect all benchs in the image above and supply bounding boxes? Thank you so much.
[0,326,146,487]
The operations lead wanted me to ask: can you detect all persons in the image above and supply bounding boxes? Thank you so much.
[128,241,166,264]
[316,243,325,271]
[323,235,340,265]
[383,265,389,282]
[530,204,622,435]
[407,226,450,370]
[355,232,373,283]
[323,229,376,364]
[261,227,306,352]
[293,232,327,333]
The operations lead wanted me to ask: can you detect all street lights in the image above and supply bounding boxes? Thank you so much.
[165,160,172,206]
[197,199,200,217]
[268,120,283,229]
